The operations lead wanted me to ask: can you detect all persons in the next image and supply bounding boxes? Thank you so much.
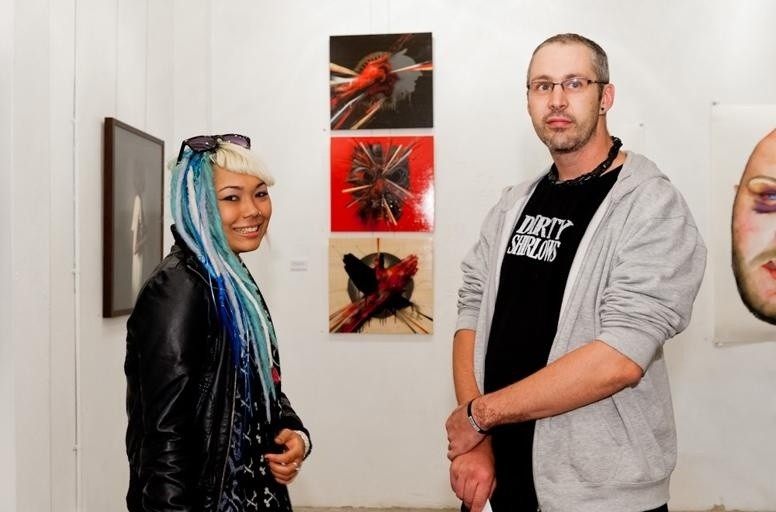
[129,158,150,311]
[443,33,709,511]
[293,461,302,471]
[122,131,313,512]
[328,252,418,335]
[729,128,776,325]
[331,53,394,130]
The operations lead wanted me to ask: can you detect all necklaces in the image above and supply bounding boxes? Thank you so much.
[547,132,625,191]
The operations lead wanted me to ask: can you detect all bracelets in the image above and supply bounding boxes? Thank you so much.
[467,396,493,437]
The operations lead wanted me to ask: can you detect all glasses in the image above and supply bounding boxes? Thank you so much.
[527,78,608,95]
[178,134,251,165]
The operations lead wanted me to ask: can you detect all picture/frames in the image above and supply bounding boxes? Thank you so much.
[101,117,165,318]
